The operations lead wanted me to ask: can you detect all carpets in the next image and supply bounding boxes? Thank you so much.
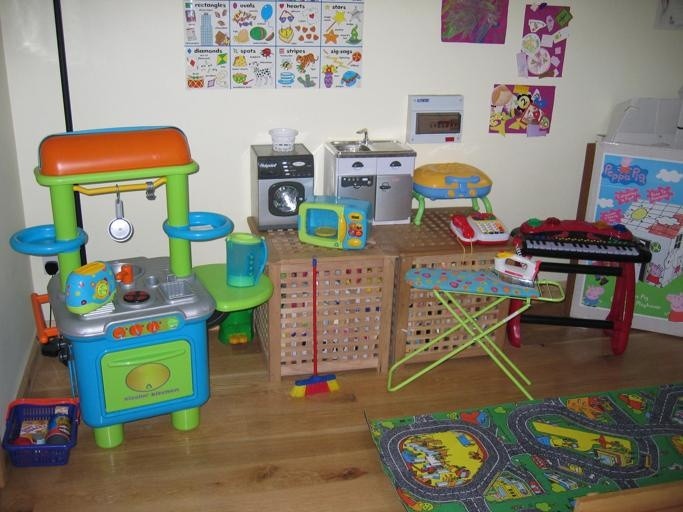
[362,379,683,512]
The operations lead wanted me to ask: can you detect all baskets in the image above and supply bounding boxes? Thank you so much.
[3,404,78,467]
